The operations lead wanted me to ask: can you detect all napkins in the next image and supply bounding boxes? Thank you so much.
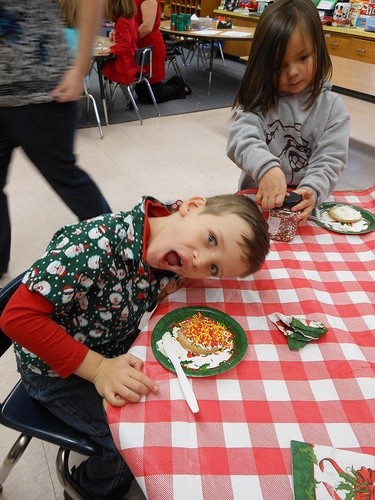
[270,312,329,355]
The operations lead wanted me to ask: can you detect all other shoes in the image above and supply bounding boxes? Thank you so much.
[172,75,192,95]
[126,100,133,110]
[63,465,77,500]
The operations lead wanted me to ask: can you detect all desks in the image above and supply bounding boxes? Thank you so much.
[90,27,114,126]
[159,21,255,95]
[103,185,375,500]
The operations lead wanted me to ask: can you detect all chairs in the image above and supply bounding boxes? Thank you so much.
[82,46,160,139]
[0,271,94,500]
[163,34,225,78]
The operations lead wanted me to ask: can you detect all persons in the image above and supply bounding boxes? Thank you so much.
[0,0,114,279]
[226,0,350,226]
[0,195,270,500]
[103,0,192,106]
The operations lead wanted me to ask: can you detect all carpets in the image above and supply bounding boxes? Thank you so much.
[85,45,248,129]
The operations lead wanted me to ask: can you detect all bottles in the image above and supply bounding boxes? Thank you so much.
[170,13,192,31]
[269,191,301,242]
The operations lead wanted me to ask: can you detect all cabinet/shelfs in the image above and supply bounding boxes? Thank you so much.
[160,0,220,21]
[215,12,375,102]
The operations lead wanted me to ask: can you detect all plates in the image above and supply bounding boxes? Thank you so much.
[312,202,375,234]
[151,305,248,377]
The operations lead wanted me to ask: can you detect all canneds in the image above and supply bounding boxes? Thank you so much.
[267,192,302,241]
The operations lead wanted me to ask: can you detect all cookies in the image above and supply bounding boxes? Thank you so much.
[329,205,361,223]
[177,312,231,354]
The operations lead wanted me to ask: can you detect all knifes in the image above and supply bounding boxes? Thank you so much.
[162,332,200,414]
